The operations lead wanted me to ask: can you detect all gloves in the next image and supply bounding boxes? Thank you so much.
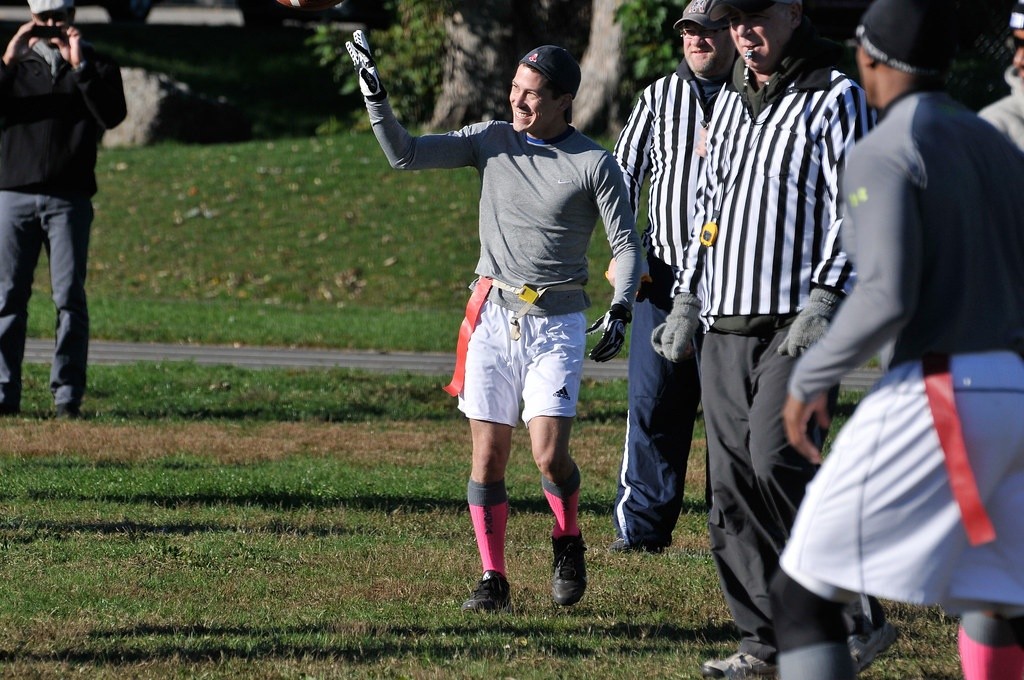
[585,304,633,362]
[777,288,842,357]
[345,30,387,102]
[651,293,702,362]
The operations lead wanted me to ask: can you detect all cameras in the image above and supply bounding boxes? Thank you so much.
[31,25,65,40]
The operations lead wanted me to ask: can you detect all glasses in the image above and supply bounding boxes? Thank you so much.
[679,28,728,39]
[1005,35,1024,55]
[33,11,67,22]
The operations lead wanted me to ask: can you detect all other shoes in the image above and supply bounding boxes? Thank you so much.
[848,621,896,674]
[611,540,667,553]
[0,403,20,415]
[57,404,80,421]
[702,652,776,680]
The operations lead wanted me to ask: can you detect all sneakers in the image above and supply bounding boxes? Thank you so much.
[461,570,513,613]
[551,532,587,605]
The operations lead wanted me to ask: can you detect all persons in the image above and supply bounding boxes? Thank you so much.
[0,0,128,419]
[651,1,899,680]
[770,0,1024,680]
[978,0,1024,152]
[604,0,712,557]
[344,31,639,612]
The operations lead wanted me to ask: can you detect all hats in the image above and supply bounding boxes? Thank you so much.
[519,45,581,123]
[1010,0,1024,29]
[710,0,776,22]
[856,0,971,76]
[27,0,74,14]
[673,0,739,31]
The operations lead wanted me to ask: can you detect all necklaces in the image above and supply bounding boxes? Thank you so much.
[741,47,765,126]
